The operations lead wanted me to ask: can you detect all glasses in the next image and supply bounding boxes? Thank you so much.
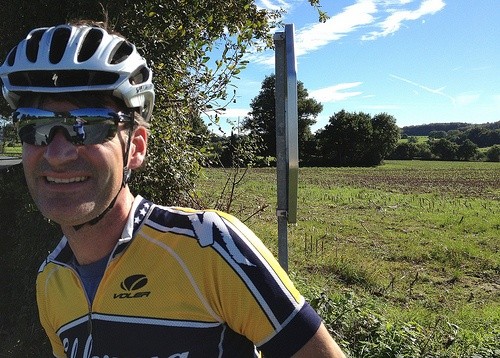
[10,104,140,142]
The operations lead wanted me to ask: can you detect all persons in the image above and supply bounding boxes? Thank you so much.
[73,115,88,145]
[1,24,350,358]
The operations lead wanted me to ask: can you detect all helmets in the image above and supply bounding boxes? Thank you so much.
[0,24,157,121]
